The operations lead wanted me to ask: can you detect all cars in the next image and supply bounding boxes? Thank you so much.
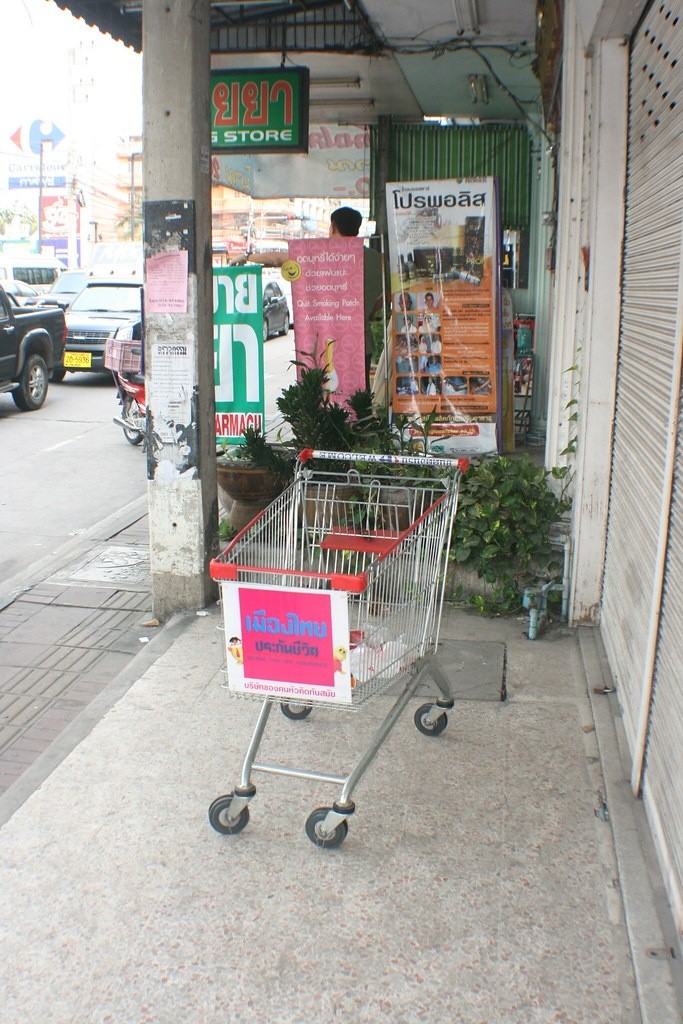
[1,270,142,382]
[262,274,295,341]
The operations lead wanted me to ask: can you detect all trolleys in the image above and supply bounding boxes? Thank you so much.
[206,441,472,854]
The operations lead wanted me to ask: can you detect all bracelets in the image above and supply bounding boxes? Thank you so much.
[244,251,253,261]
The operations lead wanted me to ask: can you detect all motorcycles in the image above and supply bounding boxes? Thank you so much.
[111,374,146,454]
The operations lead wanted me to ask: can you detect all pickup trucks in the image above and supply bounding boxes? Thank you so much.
[0,279,69,410]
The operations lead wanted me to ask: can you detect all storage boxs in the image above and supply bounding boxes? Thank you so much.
[104,339,142,374]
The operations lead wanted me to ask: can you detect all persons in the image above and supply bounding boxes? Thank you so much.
[400,314,417,333]
[429,356,441,374]
[397,293,413,312]
[419,335,428,353]
[419,314,438,333]
[426,377,437,396]
[229,206,392,402]
[430,334,442,353]
[421,293,438,312]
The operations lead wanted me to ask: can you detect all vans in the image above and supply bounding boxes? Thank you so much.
[1,253,70,297]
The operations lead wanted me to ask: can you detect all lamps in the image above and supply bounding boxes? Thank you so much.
[440,117,480,127]
[469,75,490,105]
[451,0,481,36]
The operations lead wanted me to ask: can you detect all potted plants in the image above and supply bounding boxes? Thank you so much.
[276,366,362,543]
[344,402,450,531]
[217,426,290,542]
